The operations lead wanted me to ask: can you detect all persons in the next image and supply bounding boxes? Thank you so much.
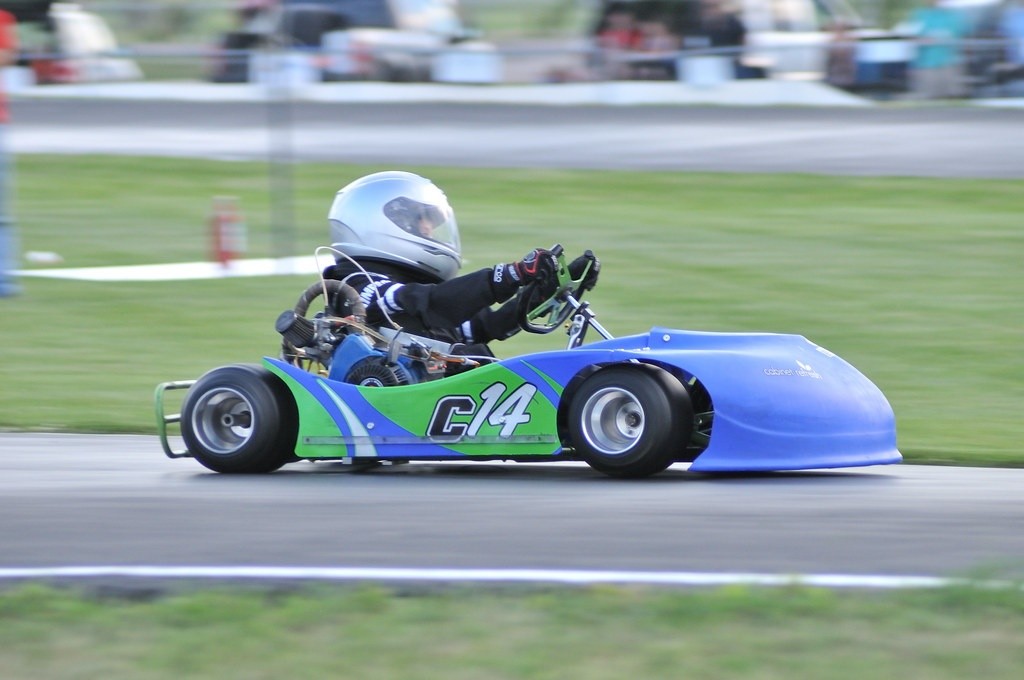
[327,171,601,379]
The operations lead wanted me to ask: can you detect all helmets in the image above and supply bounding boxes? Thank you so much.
[324,169,463,285]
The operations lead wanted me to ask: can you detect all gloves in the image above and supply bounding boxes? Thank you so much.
[492,247,556,304]
[518,249,600,318]
